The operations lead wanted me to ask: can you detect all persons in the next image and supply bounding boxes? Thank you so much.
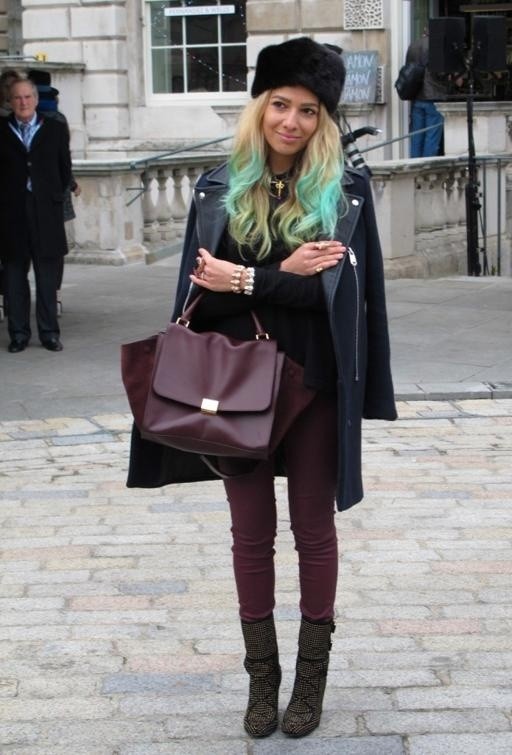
[1,76,72,352]
[173,36,379,740]
[27,69,59,111]
[404,27,463,156]
[0,70,23,117]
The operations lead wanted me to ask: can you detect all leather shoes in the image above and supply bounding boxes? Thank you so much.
[8,339,28,353]
[42,337,64,352]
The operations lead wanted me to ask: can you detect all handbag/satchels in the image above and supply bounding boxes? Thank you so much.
[120,289,316,460]
[394,63,426,102]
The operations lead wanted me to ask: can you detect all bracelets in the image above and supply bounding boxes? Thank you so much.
[230,263,245,295]
[244,265,255,297]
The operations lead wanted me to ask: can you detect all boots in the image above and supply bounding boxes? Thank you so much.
[282,616,335,738]
[241,612,282,738]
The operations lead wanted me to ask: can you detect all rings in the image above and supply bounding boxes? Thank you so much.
[315,264,324,272]
[312,241,329,250]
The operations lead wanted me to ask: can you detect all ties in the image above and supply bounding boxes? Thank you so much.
[21,123,33,193]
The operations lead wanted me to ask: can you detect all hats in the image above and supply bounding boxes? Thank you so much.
[251,38,346,117]
